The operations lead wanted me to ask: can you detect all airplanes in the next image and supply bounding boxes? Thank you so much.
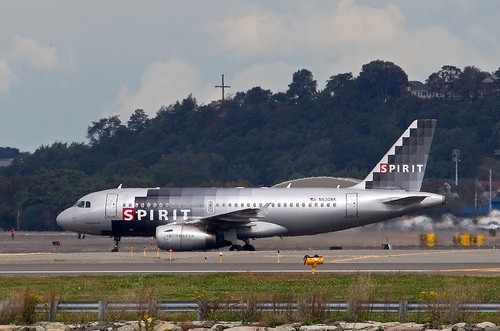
[54,118,449,259]
